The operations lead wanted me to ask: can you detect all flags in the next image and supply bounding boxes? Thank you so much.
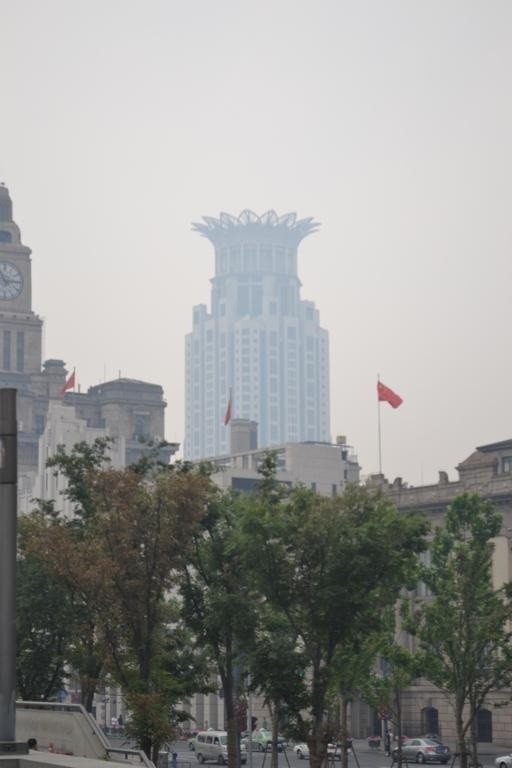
[61,373,74,396]
[223,399,232,425]
[377,381,402,407]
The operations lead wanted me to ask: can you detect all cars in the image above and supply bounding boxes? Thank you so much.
[186,736,197,750]
[495,753,512,768]
[392,738,451,764]
[293,741,351,761]
[240,728,288,753]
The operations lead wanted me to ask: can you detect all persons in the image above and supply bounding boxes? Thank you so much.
[388,729,393,743]
[384,730,391,756]
[252,716,297,734]
[111,711,133,729]
[27,738,39,751]
[426,732,438,738]
[323,731,354,752]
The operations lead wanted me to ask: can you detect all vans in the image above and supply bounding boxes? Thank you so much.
[194,730,248,765]
